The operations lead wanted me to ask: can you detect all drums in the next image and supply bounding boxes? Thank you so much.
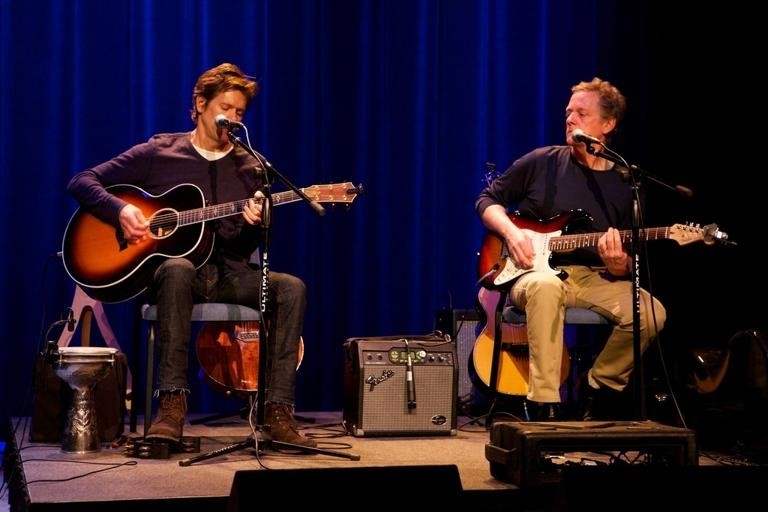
[52,347,117,454]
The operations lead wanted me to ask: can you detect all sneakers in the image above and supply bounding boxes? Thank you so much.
[146,392,187,441]
[524,396,559,421]
[576,371,605,422]
[264,402,317,454]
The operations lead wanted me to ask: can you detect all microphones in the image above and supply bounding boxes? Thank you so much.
[405,355,417,411]
[214,113,244,131]
[69,308,75,332]
[570,129,601,147]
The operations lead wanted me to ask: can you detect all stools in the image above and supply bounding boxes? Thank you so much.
[484,290,616,427]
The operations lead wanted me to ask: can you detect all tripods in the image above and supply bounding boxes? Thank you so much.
[177,152,359,466]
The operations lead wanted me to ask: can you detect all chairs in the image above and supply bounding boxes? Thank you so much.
[131,246,263,440]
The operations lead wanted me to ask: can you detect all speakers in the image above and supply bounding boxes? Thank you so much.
[436,307,488,415]
[345,338,457,438]
[229,464,464,512]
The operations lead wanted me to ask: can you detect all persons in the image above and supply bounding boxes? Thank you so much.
[474,76,668,423]
[66,62,318,454]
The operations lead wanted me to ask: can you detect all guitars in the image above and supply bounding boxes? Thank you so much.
[472,162,572,397]
[479,210,731,290]
[61,181,364,303]
